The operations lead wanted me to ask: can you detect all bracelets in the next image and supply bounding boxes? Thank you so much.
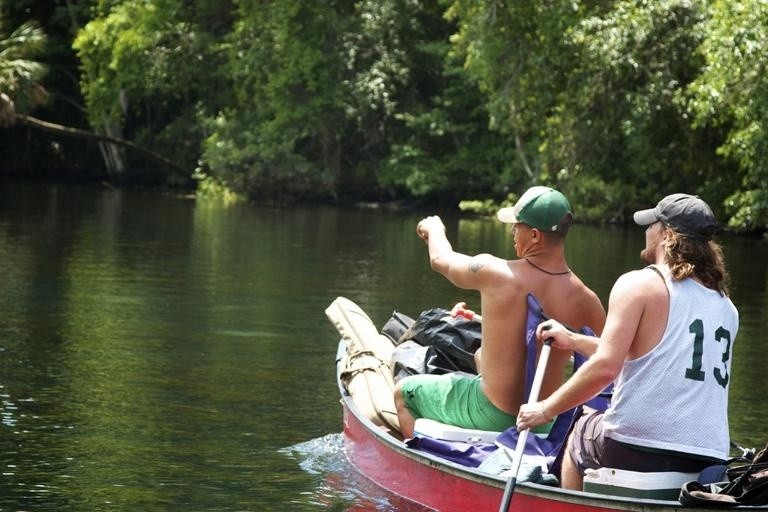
[539,399,555,424]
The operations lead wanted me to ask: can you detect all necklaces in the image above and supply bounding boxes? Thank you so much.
[525,255,572,276]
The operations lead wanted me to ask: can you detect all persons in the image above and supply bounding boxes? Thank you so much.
[515,190,739,492]
[393,184,603,440]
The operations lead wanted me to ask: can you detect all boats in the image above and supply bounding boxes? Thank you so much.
[334,338,768,512]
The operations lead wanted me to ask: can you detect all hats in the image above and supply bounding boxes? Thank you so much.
[634,192,717,238]
[497,185,573,232]
